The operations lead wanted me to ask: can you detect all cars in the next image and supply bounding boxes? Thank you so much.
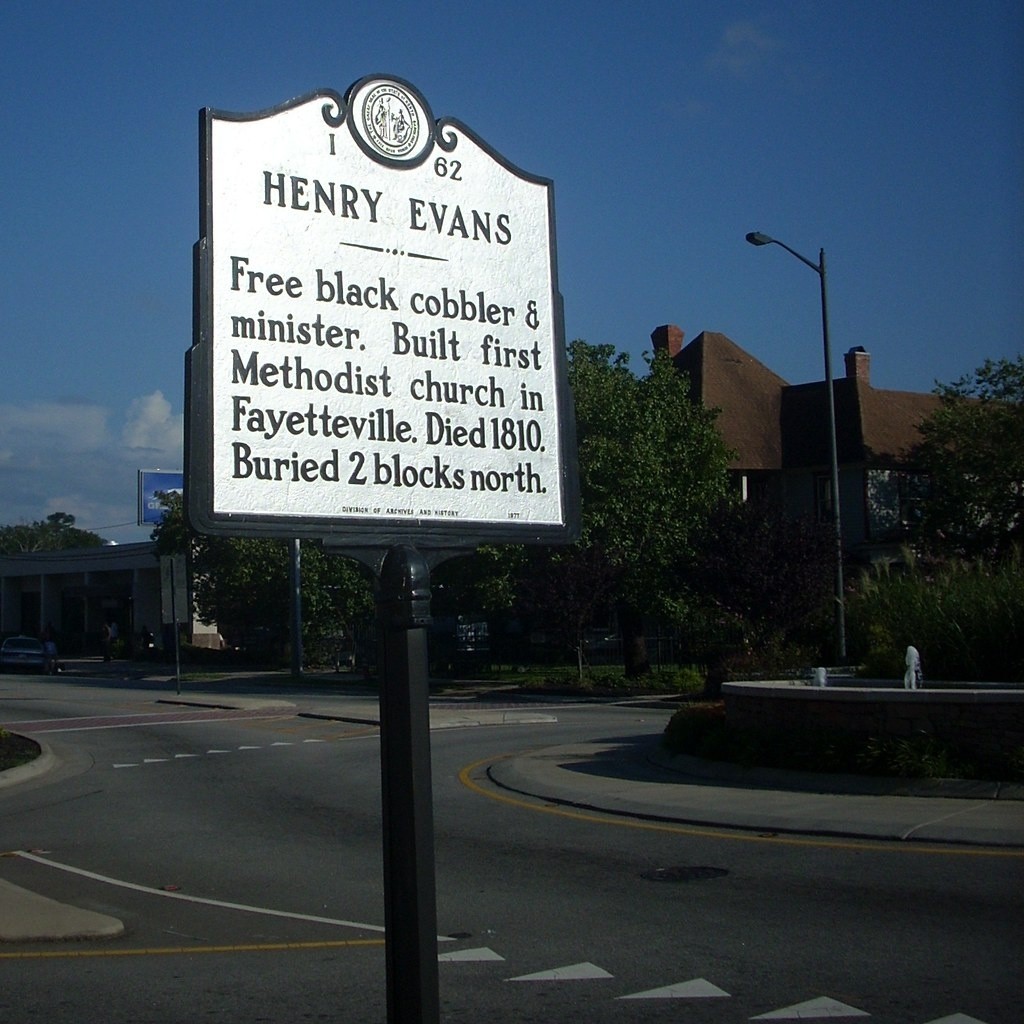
[0,634,51,674]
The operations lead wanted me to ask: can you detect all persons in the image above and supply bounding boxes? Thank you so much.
[102,617,120,663]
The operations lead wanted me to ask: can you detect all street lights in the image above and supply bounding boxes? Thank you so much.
[745,229,845,658]
